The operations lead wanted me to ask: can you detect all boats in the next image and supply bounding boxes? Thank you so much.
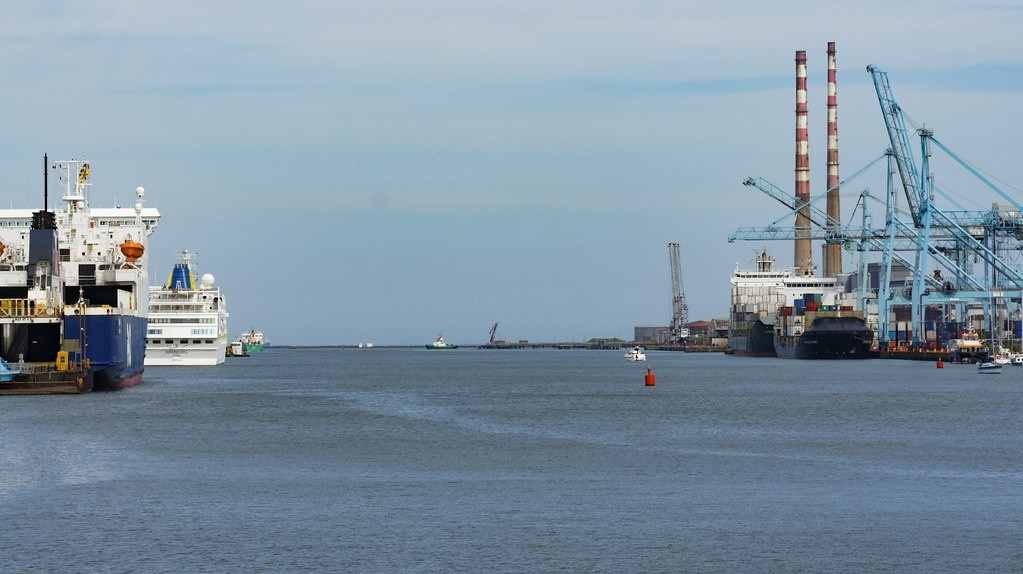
[226,329,267,358]
[424,335,459,351]
[976,355,1023,376]
[770,257,875,360]
[146,248,230,366]
[623,345,647,362]
[723,248,801,357]
[0,151,161,395]
[120,239,145,263]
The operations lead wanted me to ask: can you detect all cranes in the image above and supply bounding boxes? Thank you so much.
[666,241,690,348]
[726,62,1023,355]
